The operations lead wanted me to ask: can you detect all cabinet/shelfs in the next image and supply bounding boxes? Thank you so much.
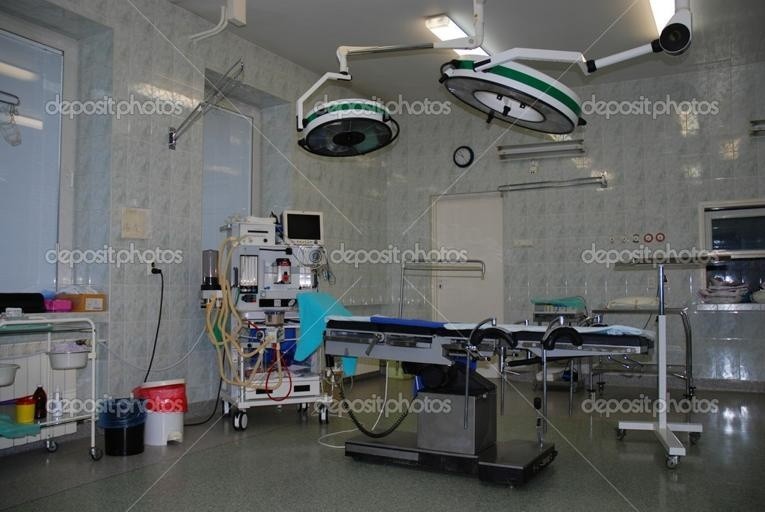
[218,306,338,433]
[586,296,695,407]
[532,298,585,395]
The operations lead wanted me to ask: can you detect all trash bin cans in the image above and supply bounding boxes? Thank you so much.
[132,379,184,446]
[104,398,145,457]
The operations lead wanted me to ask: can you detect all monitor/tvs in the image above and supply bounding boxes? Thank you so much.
[280,211,324,245]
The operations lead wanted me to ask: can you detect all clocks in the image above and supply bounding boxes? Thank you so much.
[453,145,474,168]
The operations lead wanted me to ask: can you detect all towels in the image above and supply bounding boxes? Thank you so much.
[293,291,359,378]
[0,414,42,442]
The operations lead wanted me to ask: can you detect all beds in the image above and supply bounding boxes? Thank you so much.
[324,316,657,488]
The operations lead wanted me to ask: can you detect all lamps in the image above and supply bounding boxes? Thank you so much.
[494,139,588,160]
[426,12,492,58]
[649,0,679,41]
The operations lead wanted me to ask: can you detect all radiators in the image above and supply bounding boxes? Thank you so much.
[0,316,104,462]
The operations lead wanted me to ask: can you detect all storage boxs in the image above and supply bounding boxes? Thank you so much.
[56,290,107,313]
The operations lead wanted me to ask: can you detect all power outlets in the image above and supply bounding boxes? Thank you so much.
[146,259,156,274]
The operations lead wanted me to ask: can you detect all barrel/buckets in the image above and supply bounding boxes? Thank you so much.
[16,398,37,425]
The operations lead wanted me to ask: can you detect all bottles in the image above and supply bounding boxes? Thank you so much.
[32,384,46,419]
[51,392,63,417]
